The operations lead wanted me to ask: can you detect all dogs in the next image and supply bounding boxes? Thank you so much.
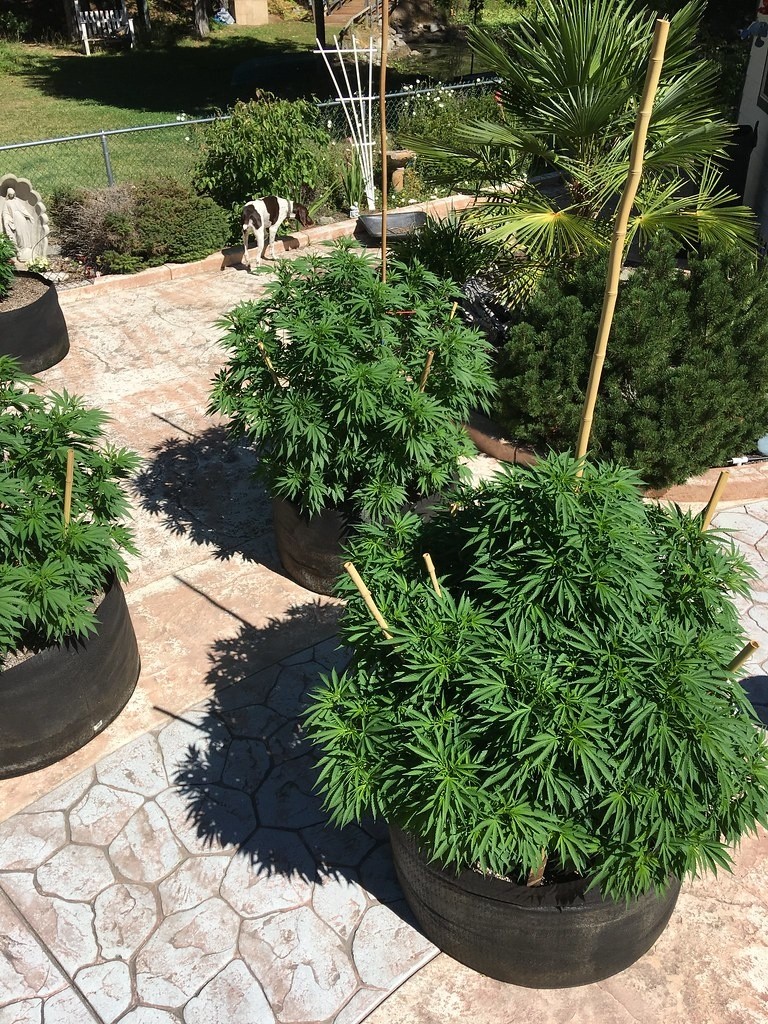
[240,196,314,268]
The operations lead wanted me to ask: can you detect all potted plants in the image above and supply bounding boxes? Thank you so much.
[0,356,141,782]
[0,235,69,381]
[207,232,501,604]
[301,437,768,991]
